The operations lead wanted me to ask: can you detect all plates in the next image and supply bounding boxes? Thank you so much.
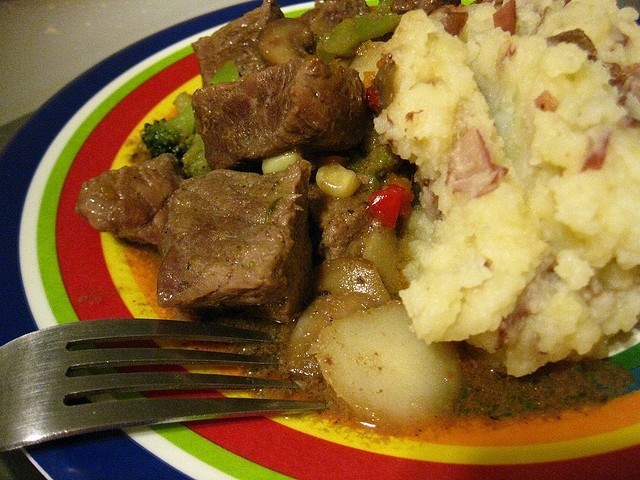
[0,0,638,480]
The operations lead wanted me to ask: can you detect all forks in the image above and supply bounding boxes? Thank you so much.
[0,317,327,453]
